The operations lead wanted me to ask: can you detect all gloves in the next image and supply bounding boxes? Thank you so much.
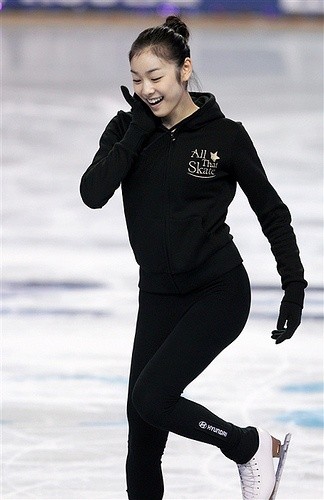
[119,85,160,134]
[270,298,302,344]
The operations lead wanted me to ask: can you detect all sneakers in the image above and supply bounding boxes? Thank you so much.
[237,426,292,500]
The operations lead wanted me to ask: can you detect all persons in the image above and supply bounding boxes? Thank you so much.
[79,16,307,500]
